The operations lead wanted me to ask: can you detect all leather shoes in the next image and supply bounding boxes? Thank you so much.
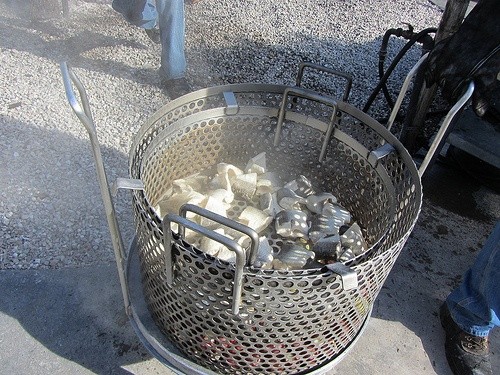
[145,22,161,44]
[166,77,191,101]
[440,300,494,375]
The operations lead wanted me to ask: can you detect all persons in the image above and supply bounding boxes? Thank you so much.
[112,0,193,99]
